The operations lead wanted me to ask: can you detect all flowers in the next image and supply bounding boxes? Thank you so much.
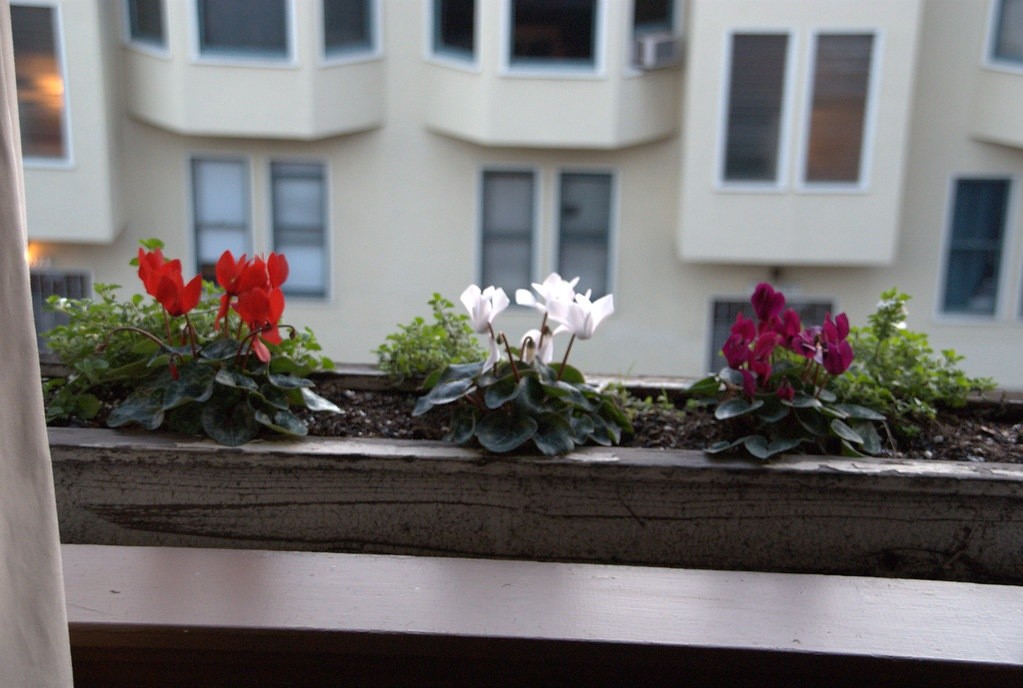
[695,282,891,465]
[410,272,634,461]
[94,246,348,450]
